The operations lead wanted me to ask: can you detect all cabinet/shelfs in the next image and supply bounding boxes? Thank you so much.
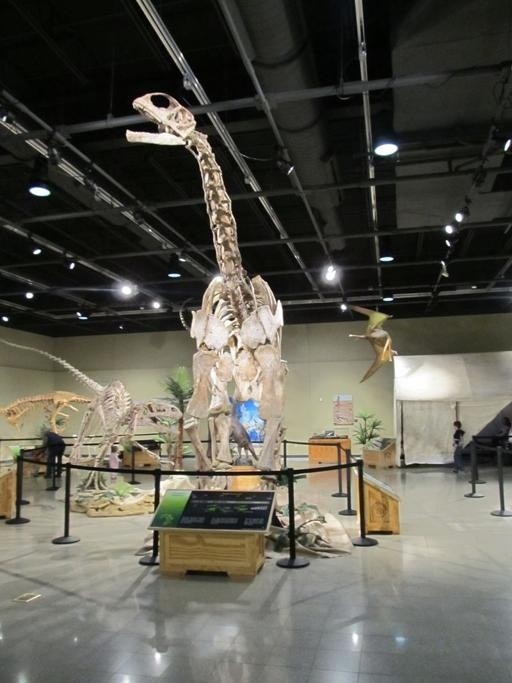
[309,438,351,464]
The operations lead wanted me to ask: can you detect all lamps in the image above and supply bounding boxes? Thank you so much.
[64,259,76,270]
[454,207,469,223]
[275,158,295,175]
[167,254,181,278]
[446,238,459,247]
[444,223,456,234]
[30,242,42,255]
[373,134,398,156]
[379,245,394,262]
[28,155,52,197]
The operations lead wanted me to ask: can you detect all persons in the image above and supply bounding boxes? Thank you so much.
[451,421,466,473]
[109,441,124,479]
[495,417,512,467]
[38,427,66,478]
